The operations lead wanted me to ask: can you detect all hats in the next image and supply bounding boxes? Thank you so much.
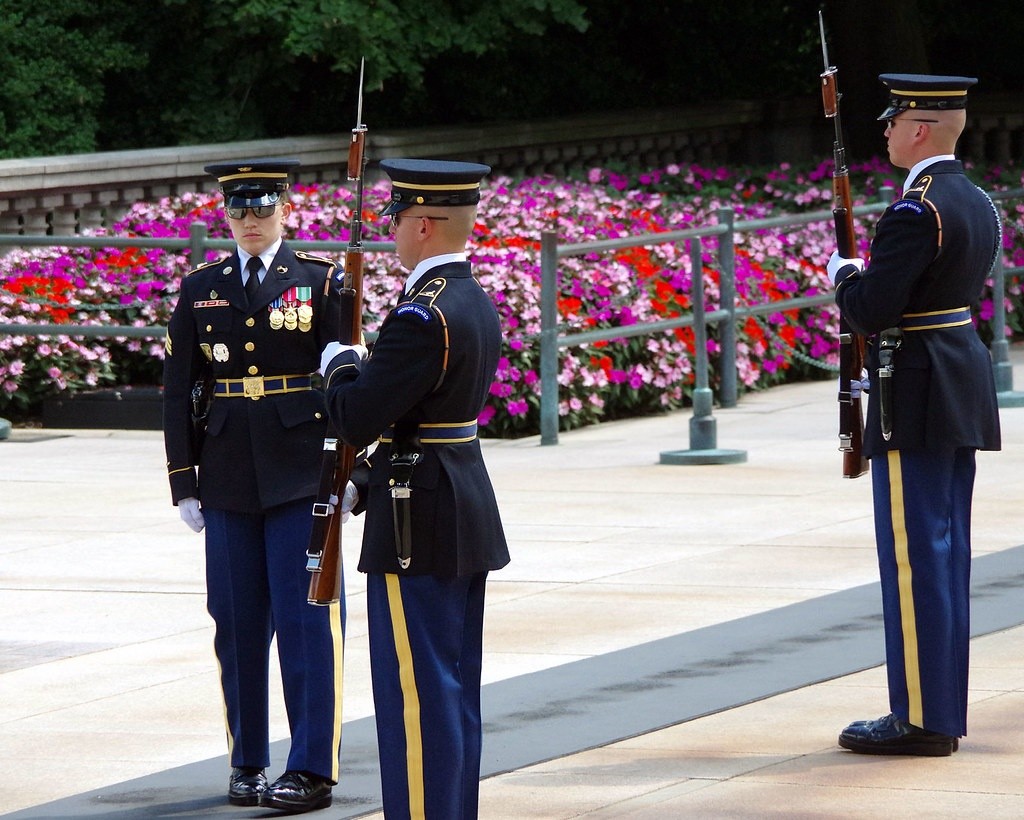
[203,158,301,211]
[376,158,492,216]
[874,73,980,121]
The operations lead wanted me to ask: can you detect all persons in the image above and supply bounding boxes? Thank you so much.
[827,74,1001,757]
[321,157,512,820]
[162,158,366,808]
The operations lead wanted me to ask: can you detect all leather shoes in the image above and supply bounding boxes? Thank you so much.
[260,771,332,813]
[227,768,269,807]
[838,713,959,757]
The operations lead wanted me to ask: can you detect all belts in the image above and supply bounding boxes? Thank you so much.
[212,375,314,397]
[379,418,479,443]
[901,306,974,330]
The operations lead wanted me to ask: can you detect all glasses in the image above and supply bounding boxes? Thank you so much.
[226,202,284,219]
[389,213,449,229]
[887,119,939,129]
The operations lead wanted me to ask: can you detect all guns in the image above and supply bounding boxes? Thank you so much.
[302,54,372,609]
[816,5,873,480]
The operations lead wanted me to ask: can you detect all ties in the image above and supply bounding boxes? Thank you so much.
[244,257,264,307]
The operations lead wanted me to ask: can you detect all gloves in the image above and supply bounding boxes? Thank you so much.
[318,341,369,377]
[826,250,867,287]
[328,479,359,524]
[837,367,871,398]
[177,497,205,533]
